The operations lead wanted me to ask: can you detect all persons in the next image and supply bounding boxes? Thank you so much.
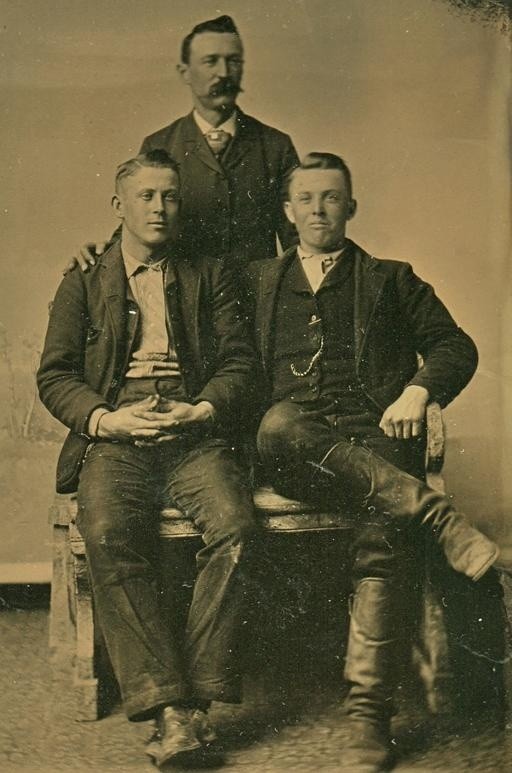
[142,17,298,259]
[40,153,268,769]
[64,152,501,772]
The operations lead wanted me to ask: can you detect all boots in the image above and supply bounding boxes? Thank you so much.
[343,577,395,773]
[311,438,500,583]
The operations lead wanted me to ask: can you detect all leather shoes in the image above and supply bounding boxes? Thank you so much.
[145,705,218,768]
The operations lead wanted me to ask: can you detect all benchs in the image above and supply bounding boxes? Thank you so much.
[49,400,455,722]
